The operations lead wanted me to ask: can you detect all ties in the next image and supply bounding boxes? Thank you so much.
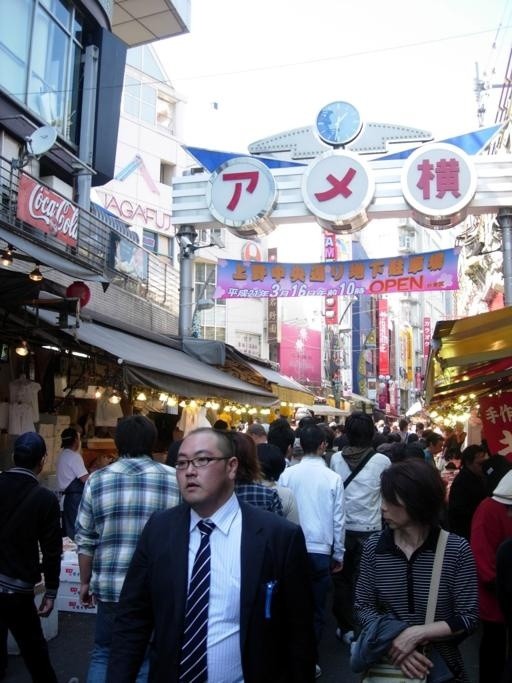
[178,521,216,683]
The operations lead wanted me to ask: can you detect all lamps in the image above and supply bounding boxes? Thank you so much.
[1,242,16,267]
[27,263,45,281]
[15,339,30,358]
[108,387,122,405]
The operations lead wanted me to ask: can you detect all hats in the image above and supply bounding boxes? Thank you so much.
[16,432,45,455]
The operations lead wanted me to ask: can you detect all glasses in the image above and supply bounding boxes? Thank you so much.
[175,457,230,469]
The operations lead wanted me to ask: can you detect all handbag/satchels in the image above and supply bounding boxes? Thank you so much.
[361,655,427,683]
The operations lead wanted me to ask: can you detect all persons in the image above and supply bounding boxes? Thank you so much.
[102,410,511,683]
[8,373,41,425]
[0,430,80,682]
[73,412,184,682]
[55,425,98,539]
